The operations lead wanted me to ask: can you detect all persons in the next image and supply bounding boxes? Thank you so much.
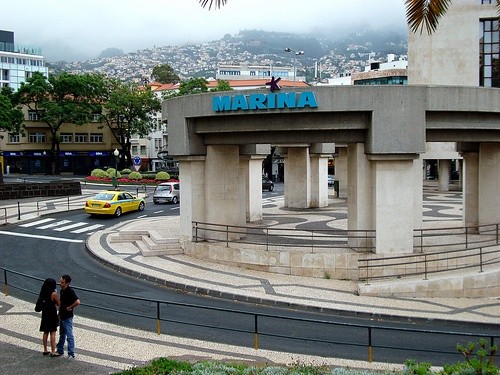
[56,275,80,359]
[34,278,60,357]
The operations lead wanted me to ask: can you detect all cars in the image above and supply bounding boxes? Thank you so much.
[262,174,275,192]
[327,174,335,187]
[152,181,179,204]
[84,188,146,218]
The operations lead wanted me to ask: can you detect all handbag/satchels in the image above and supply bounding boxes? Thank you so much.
[35,298,42,312]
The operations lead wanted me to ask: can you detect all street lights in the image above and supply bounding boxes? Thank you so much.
[113,148,120,188]
[284,47,305,87]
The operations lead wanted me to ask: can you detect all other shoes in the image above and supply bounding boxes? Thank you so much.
[51,353,60,356]
[67,355,73,359]
[43,352,49,355]
